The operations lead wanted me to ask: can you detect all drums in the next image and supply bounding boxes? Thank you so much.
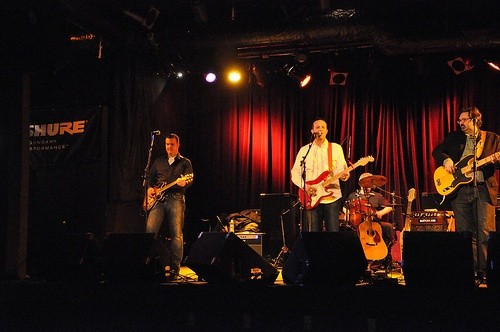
[320,222,361,238]
[339,212,364,230]
[345,196,373,219]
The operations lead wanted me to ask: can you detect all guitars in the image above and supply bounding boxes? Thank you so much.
[357,188,388,260]
[143,173,194,212]
[391,188,417,263]
[298,155,375,209]
[434,151,500,195]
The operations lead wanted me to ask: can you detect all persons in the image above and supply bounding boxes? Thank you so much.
[144,133,194,278]
[432,106,500,289]
[292,118,397,244]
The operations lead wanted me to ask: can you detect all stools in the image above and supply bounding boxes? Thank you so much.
[260,193,299,269]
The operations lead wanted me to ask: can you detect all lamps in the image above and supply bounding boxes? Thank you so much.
[166,51,348,91]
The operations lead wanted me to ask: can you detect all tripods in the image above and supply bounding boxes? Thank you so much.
[275,200,300,267]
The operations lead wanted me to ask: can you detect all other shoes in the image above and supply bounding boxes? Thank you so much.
[170,270,179,275]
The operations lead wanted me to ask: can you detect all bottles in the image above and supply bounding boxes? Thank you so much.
[230,220,234,232]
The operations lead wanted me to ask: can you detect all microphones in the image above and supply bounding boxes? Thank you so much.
[153,130,160,135]
[313,131,321,136]
[348,139,351,158]
[472,118,478,122]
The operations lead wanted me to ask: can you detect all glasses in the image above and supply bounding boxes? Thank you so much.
[457,118,472,124]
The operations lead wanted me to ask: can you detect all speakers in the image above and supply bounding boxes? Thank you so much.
[402,211,476,291]
[179,232,280,287]
[282,231,368,286]
[42,232,180,284]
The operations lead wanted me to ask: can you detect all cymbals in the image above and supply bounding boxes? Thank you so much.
[359,174,387,188]
